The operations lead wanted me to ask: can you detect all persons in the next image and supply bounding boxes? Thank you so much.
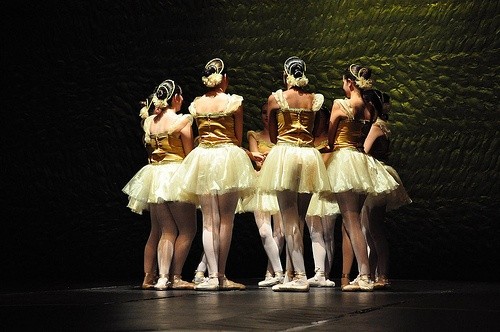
[122,57,412,291]
